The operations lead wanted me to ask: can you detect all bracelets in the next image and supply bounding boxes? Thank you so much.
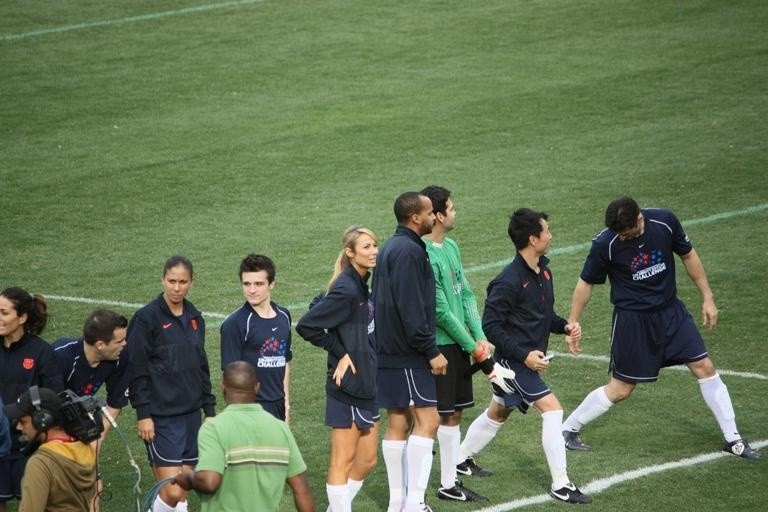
[474,351,485,360]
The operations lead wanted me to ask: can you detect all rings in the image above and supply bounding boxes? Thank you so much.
[534,364,537,368]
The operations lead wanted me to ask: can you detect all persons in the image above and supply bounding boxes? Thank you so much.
[171,360,315,512]
[419,186,515,504]
[220,254,292,427]
[117,257,216,511]
[0,286,128,511]
[563,197,762,462]
[296,226,381,512]
[369,192,448,512]
[454,208,592,505]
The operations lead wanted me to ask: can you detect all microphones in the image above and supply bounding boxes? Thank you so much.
[19,433,39,453]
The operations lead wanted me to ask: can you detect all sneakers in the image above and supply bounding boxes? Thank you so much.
[550,483,592,503]
[561,429,590,451]
[387,503,433,512]
[722,437,763,460]
[437,457,493,502]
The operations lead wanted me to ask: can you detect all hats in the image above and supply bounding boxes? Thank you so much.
[2,384,60,419]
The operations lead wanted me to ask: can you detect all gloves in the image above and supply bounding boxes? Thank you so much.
[482,357,516,396]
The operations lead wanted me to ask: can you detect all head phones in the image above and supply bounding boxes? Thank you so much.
[30,386,54,431]
